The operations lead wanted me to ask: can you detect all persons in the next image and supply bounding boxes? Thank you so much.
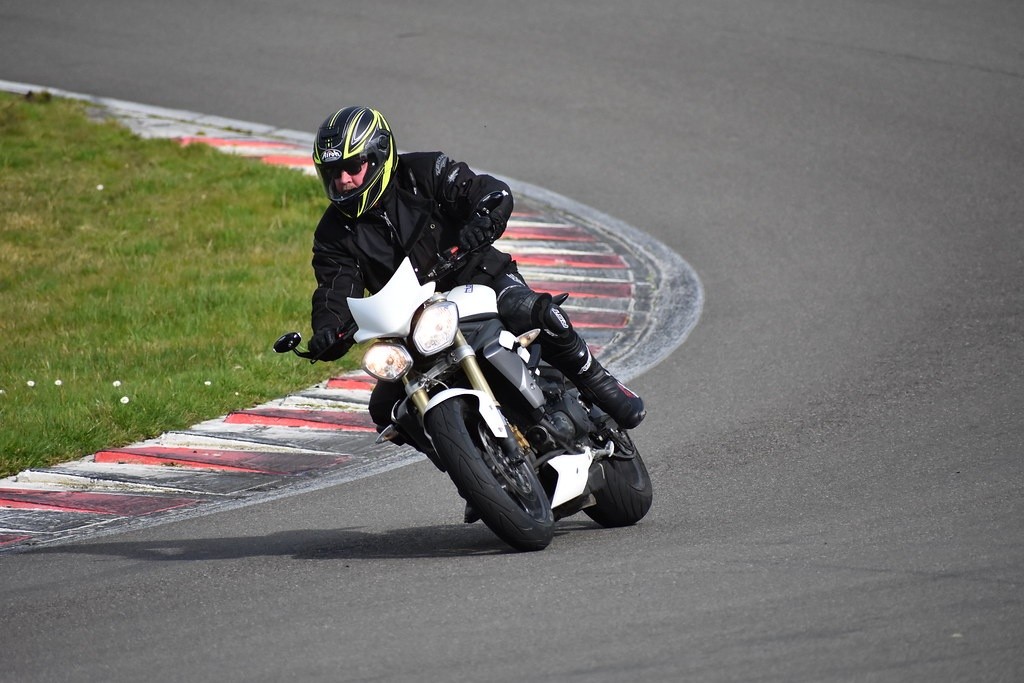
[308,104,647,524]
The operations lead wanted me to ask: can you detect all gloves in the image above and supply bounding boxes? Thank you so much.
[306,327,336,357]
[456,211,507,252]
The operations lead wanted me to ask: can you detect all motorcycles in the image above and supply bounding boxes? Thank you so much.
[273,189,654,553]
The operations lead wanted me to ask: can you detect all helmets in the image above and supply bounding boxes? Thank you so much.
[311,105,399,219]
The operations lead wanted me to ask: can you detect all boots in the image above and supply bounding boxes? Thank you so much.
[543,329,647,429]
[462,501,481,523]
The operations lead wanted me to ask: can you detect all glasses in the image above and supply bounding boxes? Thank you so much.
[330,157,369,178]
